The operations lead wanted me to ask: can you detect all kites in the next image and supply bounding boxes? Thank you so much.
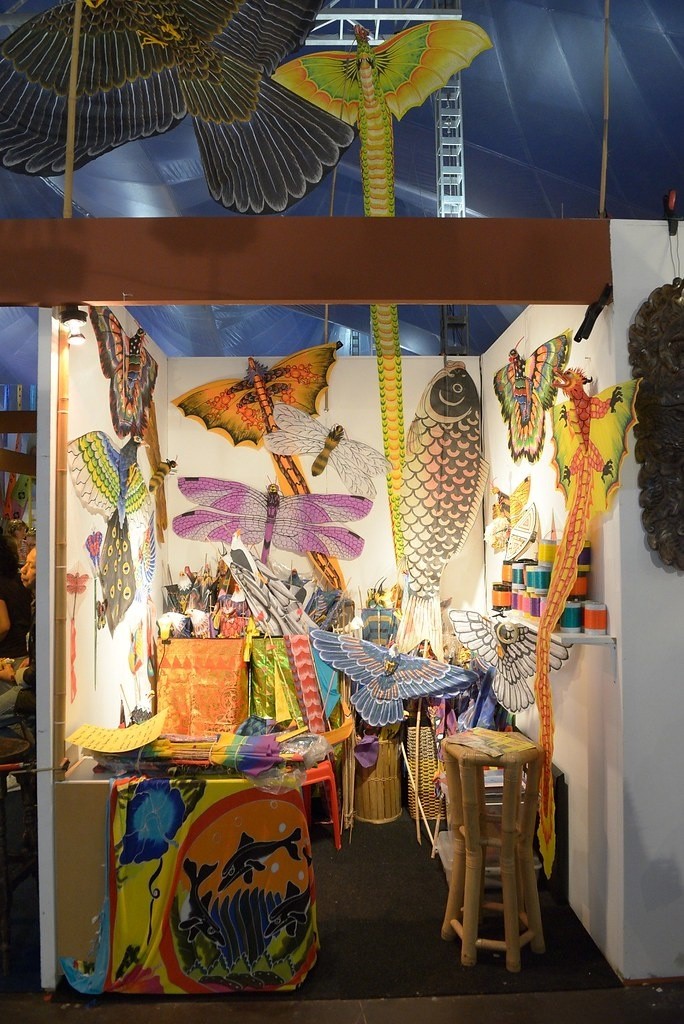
[272,21,494,217]
[0,0,358,213]
[65,306,651,882]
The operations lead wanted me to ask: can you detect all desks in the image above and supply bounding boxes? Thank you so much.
[55,756,319,992]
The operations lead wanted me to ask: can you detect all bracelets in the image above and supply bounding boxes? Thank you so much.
[10,672,17,686]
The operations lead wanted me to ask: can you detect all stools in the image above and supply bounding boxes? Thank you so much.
[298,760,342,850]
[440,732,546,972]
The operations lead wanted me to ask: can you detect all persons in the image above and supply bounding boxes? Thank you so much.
[0,519,38,664]
[0,547,36,741]
